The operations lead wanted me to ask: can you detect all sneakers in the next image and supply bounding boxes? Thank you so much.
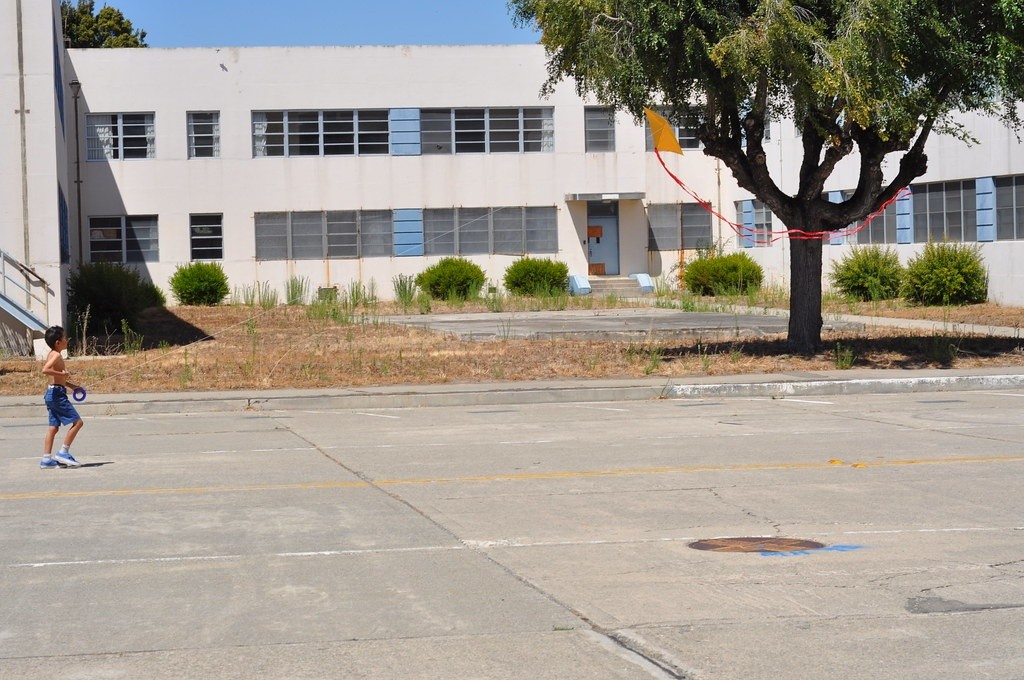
[55,452,81,467]
[40,459,66,468]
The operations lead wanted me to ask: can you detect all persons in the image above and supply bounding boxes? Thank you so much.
[39,325,83,469]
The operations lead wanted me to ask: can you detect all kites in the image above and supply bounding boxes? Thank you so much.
[642,105,683,156]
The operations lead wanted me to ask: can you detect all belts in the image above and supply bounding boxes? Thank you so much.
[48,385,62,389]
